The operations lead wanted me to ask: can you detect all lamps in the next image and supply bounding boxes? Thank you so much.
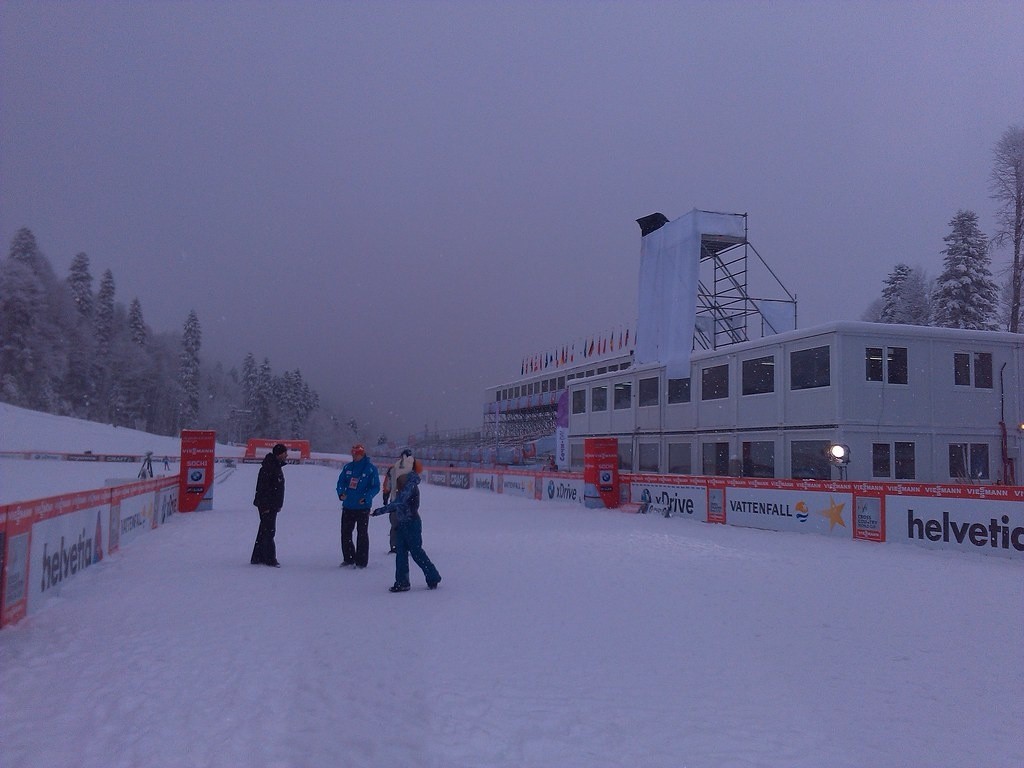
[824,443,850,480]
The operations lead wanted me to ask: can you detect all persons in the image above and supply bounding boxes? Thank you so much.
[336,443,423,569]
[370,456,441,592]
[161,456,170,471]
[250,444,290,568]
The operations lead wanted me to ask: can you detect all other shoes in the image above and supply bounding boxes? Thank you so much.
[389,585,410,592]
[340,561,353,567]
[267,561,280,568]
[427,576,441,590]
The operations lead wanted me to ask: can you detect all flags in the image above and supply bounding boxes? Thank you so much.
[571,344,574,362]
[624,330,629,346]
[560,345,564,366]
[589,340,595,356]
[550,353,553,366]
[544,353,548,367]
[602,339,606,354]
[598,338,600,355]
[584,339,588,358]
[634,330,637,345]
[610,332,613,351]
[555,350,558,368]
[521,353,543,375]
[618,332,622,349]
[565,346,568,362]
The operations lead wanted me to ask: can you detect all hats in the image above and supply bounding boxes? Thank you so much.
[272,444,288,455]
[350,444,364,456]
[400,449,412,458]
[395,456,414,479]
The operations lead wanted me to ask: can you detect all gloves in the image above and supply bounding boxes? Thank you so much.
[383,494,388,505]
